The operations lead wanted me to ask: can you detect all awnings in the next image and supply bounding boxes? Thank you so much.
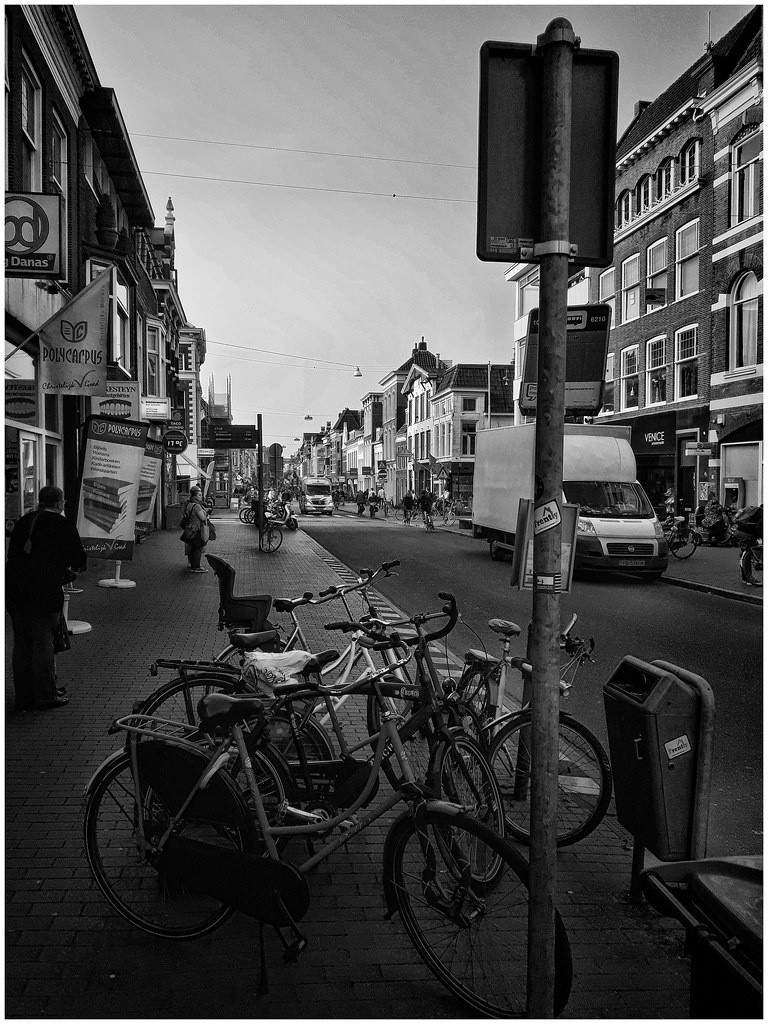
[592,403,710,455]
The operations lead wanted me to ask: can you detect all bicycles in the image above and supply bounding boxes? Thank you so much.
[358,498,461,533]
[660,515,698,560]
[79,559,615,1019]
[239,496,298,530]
[259,509,284,554]
[738,538,763,589]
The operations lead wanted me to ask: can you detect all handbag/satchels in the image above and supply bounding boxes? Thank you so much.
[180,503,204,549]
[200,518,210,543]
[54,613,71,652]
[179,516,189,530]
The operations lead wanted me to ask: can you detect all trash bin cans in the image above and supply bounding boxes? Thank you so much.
[636,856,763,1019]
[600,655,700,866]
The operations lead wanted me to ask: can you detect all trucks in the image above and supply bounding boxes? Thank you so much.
[297,476,335,516]
[464,421,669,583]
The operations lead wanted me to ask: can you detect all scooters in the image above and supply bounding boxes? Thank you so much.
[692,505,745,549]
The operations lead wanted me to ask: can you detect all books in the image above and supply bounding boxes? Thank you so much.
[83,477,135,534]
[136,480,156,515]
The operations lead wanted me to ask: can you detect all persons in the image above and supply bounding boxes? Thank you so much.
[277,490,298,503]
[5,487,87,709]
[234,485,250,494]
[269,489,276,501]
[332,490,345,510]
[354,487,385,517]
[664,488,675,519]
[735,504,763,586]
[185,483,210,573]
[401,487,450,531]
[702,491,726,541]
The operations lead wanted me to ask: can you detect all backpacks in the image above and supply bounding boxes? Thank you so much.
[422,496,430,507]
[727,505,763,540]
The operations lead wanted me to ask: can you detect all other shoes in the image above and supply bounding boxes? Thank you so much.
[32,696,69,710]
[56,688,68,696]
[190,565,209,572]
[14,698,30,710]
[742,575,762,586]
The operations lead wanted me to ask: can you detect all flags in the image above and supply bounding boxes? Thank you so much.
[36,266,108,398]
[414,459,422,474]
[429,452,437,467]
